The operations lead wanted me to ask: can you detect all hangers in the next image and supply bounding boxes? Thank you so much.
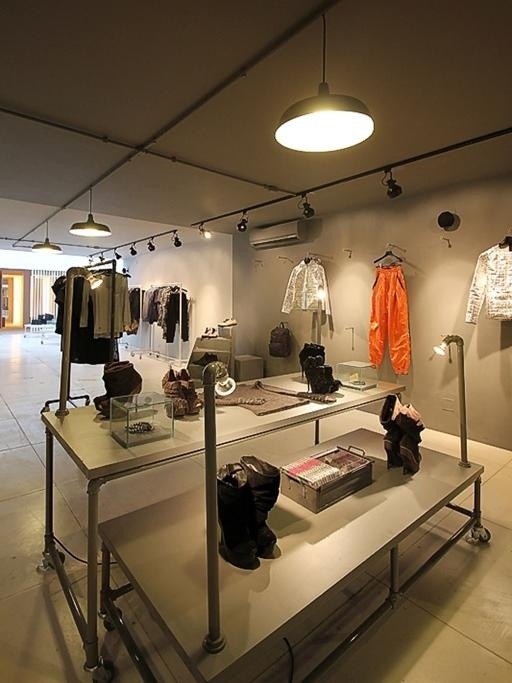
[151,283,189,295]
[304,252,313,265]
[498,230,512,249]
[83,260,131,278]
[373,242,407,264]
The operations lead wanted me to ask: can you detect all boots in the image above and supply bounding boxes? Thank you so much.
[217,462,260,571]
[379,394,404,470]
[395,403,425,474]
[239,455,281,557]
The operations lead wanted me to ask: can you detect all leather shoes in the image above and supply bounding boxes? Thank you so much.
[208,328,219,339]
[190,350,219,366]
[218,317,237,327]
[202,327,212,338]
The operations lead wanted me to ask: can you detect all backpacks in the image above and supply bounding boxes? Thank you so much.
[268,322,290,357]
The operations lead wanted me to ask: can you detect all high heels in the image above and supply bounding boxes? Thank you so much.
[161,367,187,417]
[93,360,143,419]
[298,343,325,380]
[322,364,341,389]
[177,369,204,415]
[304,366,338,395]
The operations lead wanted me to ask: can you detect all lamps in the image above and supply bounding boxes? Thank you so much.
[147,238,157,252]
[380,167,405,200]
[32,220,62,255]
[274,15,376,154]
[113,248,123,261]
[129,244,138,256]
[431,334,469,467]
[235,212,252,234]
[203,362,238,655]
[99,252,106,262]
[88,256,95,264]
[170,232,182,247]
[197,222,214,240]
[298,194,317,220]
[68,189,112,238]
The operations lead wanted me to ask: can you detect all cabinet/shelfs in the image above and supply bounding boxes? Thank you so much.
[37,365,492,683]
